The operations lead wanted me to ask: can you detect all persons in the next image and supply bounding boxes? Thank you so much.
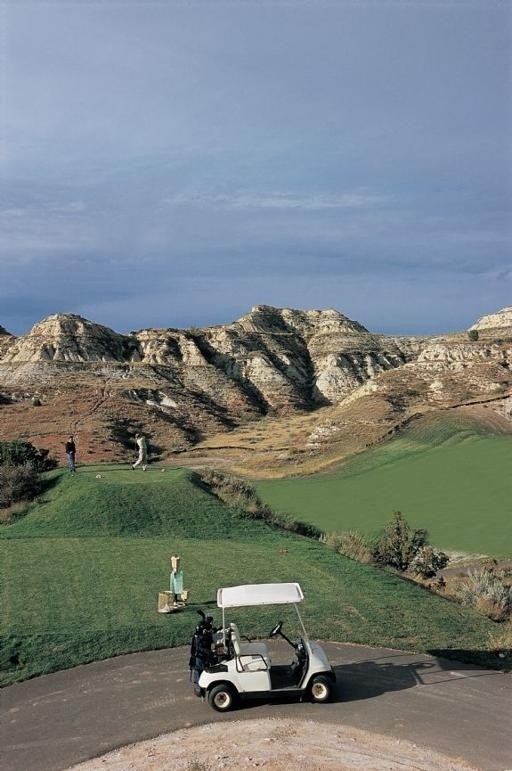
[189,616,223,684]
[131,433,148,471]
[66,436,76,477]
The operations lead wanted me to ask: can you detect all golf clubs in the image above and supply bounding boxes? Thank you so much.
[134,439,139,453]
